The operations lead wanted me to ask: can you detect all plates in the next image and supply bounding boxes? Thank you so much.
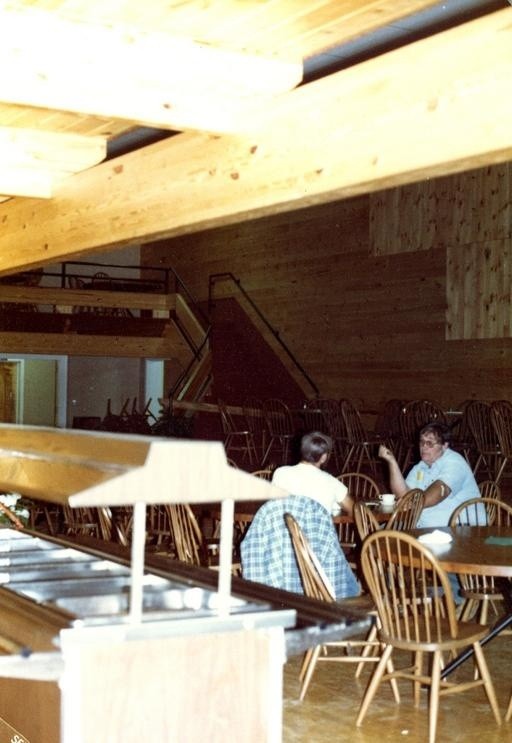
[418,533,453,546]
[380,501,396,506]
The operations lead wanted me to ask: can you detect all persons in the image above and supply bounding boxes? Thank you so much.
[269,430,354,519]
[377,423,488,621]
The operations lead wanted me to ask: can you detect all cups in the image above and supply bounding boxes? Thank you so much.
[382,506,393,514]
[379,494,395,503]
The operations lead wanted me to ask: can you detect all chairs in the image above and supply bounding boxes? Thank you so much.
[161,505,244,579]
[354,530,504,741]
[239,495,362,657]
[281,513,404,705]
[22,396,512,559]
[447,497,510,682]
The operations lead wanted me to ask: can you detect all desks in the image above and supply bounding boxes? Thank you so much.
[354,525,512,680]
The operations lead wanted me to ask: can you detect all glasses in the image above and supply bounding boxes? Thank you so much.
[419,441,438,448]
